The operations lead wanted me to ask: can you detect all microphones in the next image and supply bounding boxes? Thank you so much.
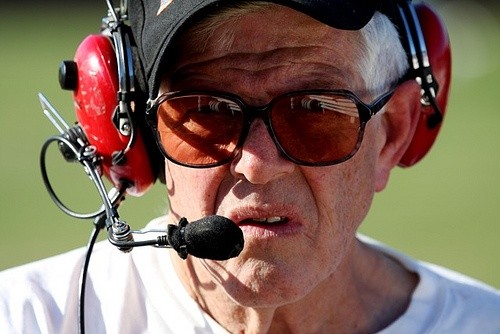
[58,126,244,262]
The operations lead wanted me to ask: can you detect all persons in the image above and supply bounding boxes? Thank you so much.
[0,0,500,334]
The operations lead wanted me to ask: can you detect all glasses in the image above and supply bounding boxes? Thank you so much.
[145,82,399,170]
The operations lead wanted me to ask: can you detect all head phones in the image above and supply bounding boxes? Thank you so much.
[56,0,454,196]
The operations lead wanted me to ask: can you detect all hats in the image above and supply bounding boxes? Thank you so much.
[124,0,388,100]
